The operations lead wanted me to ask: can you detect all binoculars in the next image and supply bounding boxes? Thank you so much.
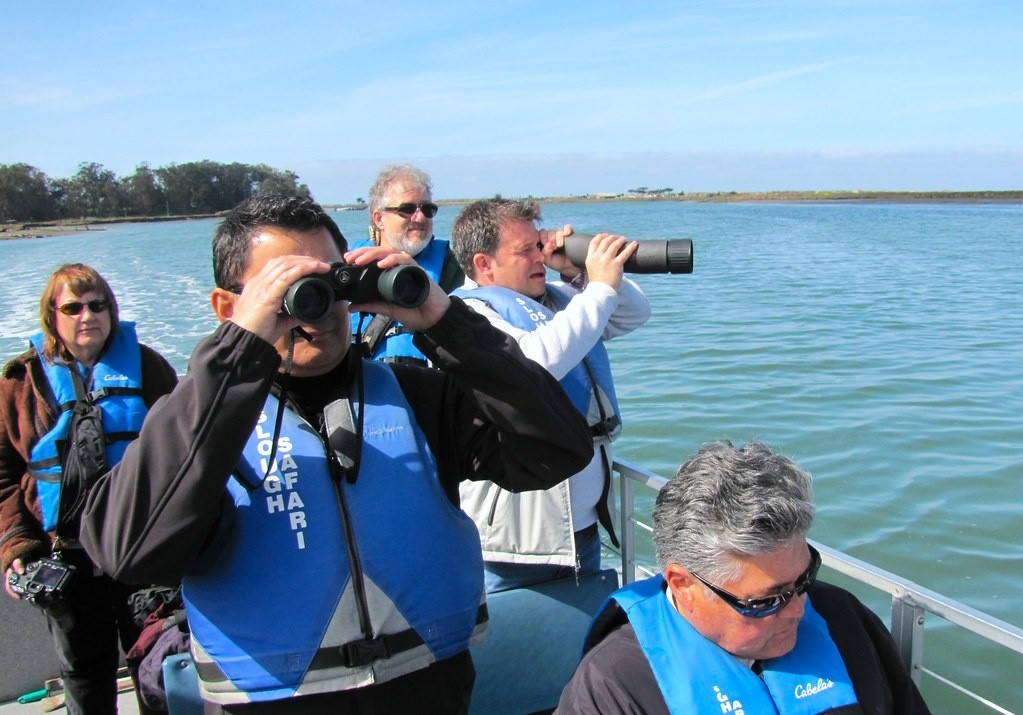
[280,261,430,324]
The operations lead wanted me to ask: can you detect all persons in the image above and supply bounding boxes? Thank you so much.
[420,194,652,599]
[555,438,932,715]
[78,196,595,715]
[0,260,180,715]
[347,166,462,363]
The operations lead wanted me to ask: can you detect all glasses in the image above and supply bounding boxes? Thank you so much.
[53,300,110,316]
[382,202,438,219]
[690,543,821,619]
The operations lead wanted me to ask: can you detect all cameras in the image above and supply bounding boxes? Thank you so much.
[7,554,85,632]
